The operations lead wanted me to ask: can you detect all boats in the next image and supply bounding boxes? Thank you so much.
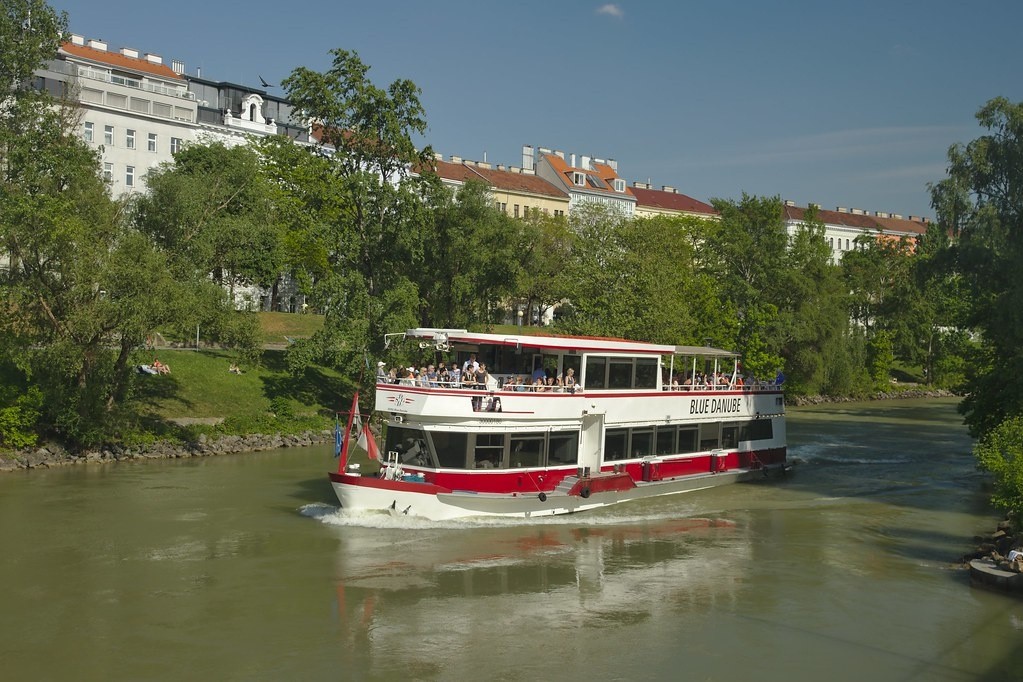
[328,328,798,522]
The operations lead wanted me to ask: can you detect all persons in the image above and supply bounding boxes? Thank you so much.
[376,351,583,396]
[659,368,782,392]
